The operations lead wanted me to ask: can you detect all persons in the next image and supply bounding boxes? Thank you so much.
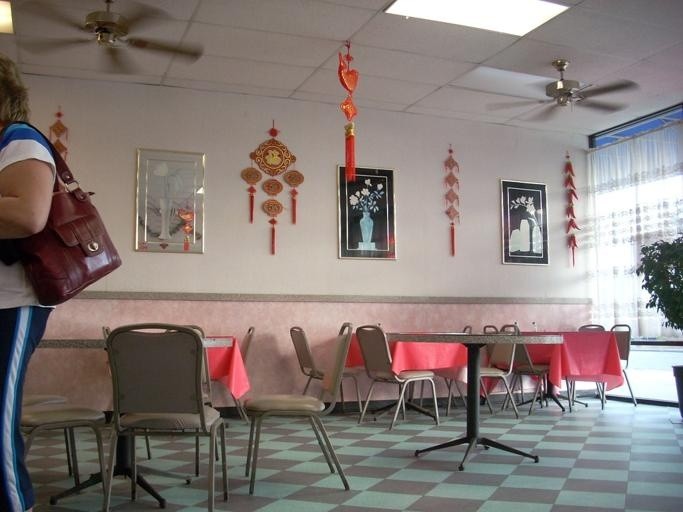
[0,52,63,511]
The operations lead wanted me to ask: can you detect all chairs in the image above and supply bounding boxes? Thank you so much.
[106,322,230,512]
[243,319,355,497]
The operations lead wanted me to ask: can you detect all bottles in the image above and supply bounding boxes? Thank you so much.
[531,322,537,332]
[514,321,519,328]
[519,218,531,252]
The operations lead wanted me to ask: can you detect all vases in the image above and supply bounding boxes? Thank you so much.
[508,219,542,255]
[359,211,373,243]
[156,197,173,240]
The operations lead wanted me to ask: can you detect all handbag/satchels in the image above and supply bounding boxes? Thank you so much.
[0,121,122,307]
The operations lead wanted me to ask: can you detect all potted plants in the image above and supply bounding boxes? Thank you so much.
[631,230,682,420]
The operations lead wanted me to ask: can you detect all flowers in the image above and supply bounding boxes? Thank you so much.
[348,178,385,215]
[507,195,538,224]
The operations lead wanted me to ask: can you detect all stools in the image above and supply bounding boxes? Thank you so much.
[18,392,74,478]
[18,407,110,512]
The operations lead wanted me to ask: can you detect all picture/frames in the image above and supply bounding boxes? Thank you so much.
[130,146,208,255]
[335,164,396,262]
[497,178,551,267]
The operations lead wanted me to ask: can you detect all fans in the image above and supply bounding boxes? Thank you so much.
[8,0,208,80]
[477,59,641,125]
